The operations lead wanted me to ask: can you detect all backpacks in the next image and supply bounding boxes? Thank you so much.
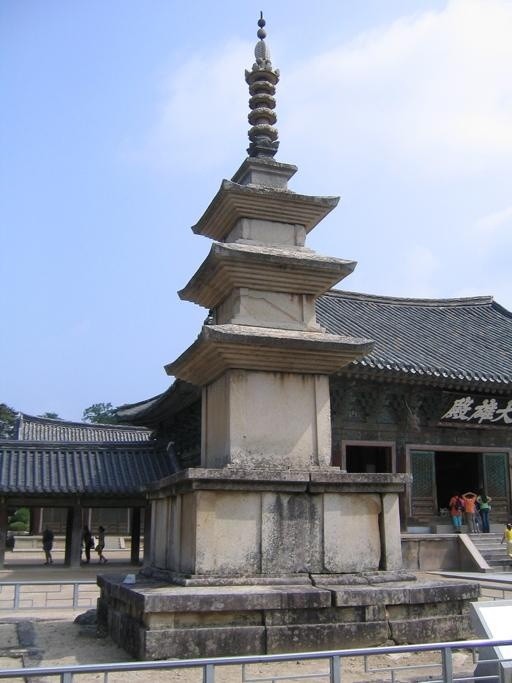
[454,496,465,512]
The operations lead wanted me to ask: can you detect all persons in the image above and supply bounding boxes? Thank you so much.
[501,523,512,558]
[83,525,91,563]
[449,488,492,533]
[95,526,108,564]
[42,524,54,565]
